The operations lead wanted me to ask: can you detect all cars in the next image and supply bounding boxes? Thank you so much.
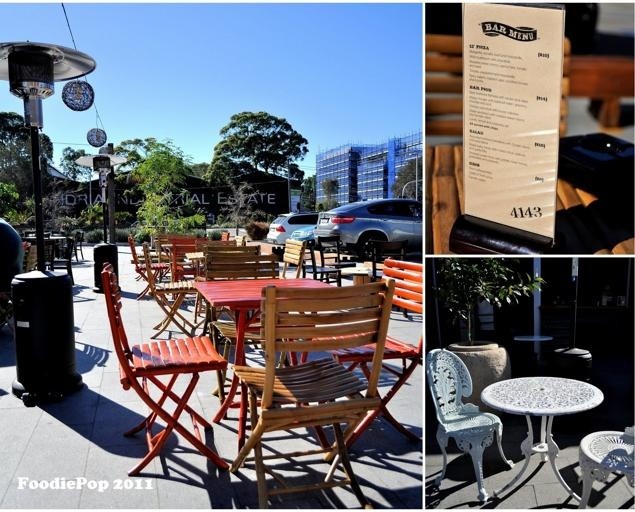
[291,226,316,245]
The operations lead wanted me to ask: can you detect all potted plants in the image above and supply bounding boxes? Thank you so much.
[437,258,545,412]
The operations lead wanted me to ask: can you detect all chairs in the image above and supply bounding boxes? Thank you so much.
[100,263,230,473]
[221,231,229,241]
[129,235,169,301]
[143,242,198,338]
[578,425,635,509]
[194,245,260,323]
[301,239,341,287]
[52,230,65,237]
[23,237,54,271]
[0,245,36,338]
[318,235,354,287]
[230,278,394,509]
[197,241,235,264]
[282,238,307,278]
[331,256,423,452]
[74,232,85,262]
[170,238,208,298]
[236,234,247,246]
[157,236,169,283]
[25,231,36,237]
[44,233,51,239]
[130,234,157,281]
[426,35,568,144]
[206,255,288,420]
[49,238,74,286]
[427,351,513,502]
[369,240,408,317]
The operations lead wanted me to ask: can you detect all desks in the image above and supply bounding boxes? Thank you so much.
[341,267,369,286]
[481,377,604,502]
[425,147,634,254]
[27,236,71,241]
[173,260,279,276]
[195,277,331,464]
[162,243,195,252]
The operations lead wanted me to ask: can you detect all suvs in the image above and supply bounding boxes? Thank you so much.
[317,197,423,261]
[267,212,319,245]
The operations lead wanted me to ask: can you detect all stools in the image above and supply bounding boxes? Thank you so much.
[567,56,635,125]
